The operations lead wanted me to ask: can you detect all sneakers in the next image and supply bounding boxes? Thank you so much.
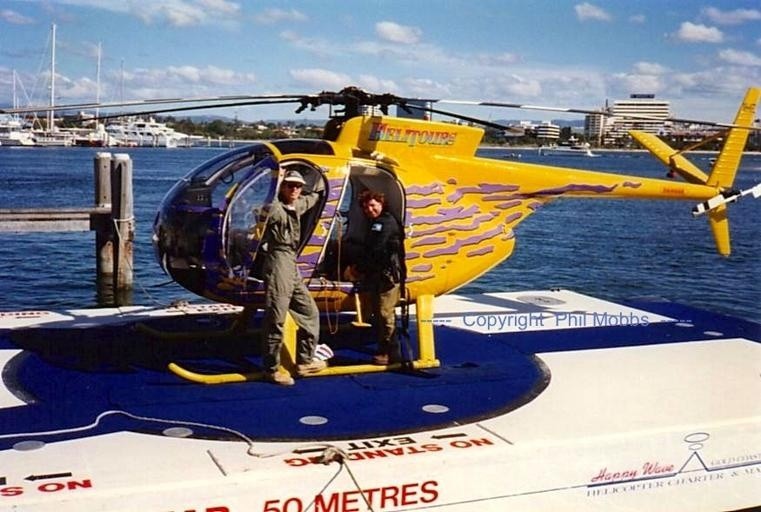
[296,359,328,376]
[262,368,295,385]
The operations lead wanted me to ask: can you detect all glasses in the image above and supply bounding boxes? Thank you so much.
[286,181,305,189]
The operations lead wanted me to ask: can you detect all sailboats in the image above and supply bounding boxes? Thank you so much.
[0,25,204,150]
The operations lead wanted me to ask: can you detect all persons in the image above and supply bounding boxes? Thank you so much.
[243,170,328,386]
[343,191,403,366]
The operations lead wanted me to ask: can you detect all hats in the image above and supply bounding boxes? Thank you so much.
[283,171,307,186]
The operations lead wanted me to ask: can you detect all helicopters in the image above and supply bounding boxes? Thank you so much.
[0,86,761,388]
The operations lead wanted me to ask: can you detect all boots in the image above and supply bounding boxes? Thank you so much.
[371,344,393,364]
